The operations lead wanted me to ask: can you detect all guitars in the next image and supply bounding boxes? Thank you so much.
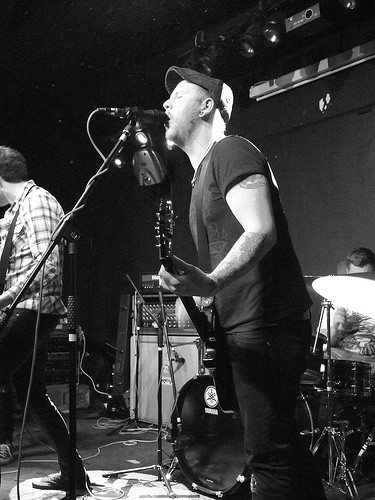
[155,197,239,413]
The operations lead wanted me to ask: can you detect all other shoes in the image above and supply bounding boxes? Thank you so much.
[32,466,91,496]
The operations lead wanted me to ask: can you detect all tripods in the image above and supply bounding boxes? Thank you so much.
[101,326,177,498]
[306,307,360,500]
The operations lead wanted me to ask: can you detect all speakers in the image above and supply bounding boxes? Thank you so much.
[130,335,203,429]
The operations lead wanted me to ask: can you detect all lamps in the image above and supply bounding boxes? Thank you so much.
[200,0,366,76]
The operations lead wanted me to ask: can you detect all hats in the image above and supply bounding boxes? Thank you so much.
[164,66,233,124]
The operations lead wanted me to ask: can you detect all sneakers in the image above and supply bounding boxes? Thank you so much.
[0,441,14,466]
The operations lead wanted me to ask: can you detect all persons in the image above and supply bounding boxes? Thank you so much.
[157,66,327,500]
[0,145,92,493]
[320,247,375,390]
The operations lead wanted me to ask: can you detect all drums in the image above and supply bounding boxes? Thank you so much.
[313,358,372,396]
[299,330,328,384]
[170,375,315,497]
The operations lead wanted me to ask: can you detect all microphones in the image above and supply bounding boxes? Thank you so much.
[97,107,169,125]
[171,358,186,363]
